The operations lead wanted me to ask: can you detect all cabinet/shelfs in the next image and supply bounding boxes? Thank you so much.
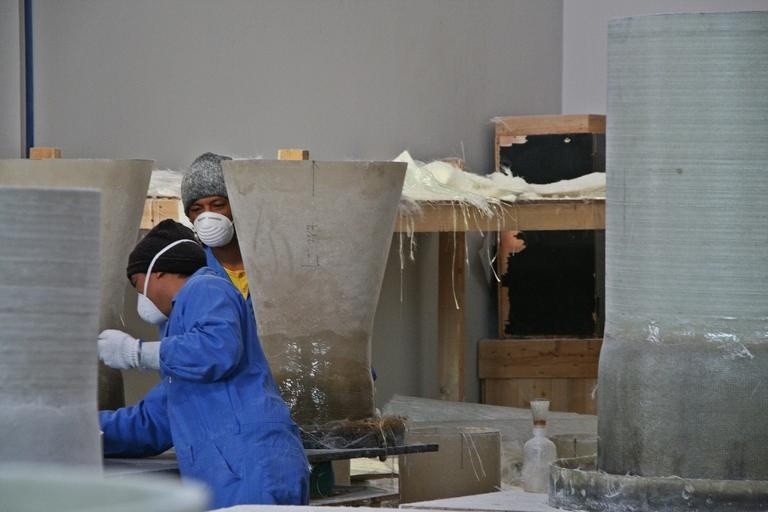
[104,443,439,507]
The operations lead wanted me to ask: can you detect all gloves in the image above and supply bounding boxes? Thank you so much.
[96,330,141,371]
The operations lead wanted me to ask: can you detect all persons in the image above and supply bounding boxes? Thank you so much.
[180,151,248,302]
[96,218,309,512]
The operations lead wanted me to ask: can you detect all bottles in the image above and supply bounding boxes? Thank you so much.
[521,429,558,493]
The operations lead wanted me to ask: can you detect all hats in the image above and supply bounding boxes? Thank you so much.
[179,153,233,217]
[127,219,207,275]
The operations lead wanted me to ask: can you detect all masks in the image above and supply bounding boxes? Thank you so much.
[194,210,235,248]
[136,292,172,323]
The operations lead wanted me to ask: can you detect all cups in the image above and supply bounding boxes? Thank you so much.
[528,399,550,429]
